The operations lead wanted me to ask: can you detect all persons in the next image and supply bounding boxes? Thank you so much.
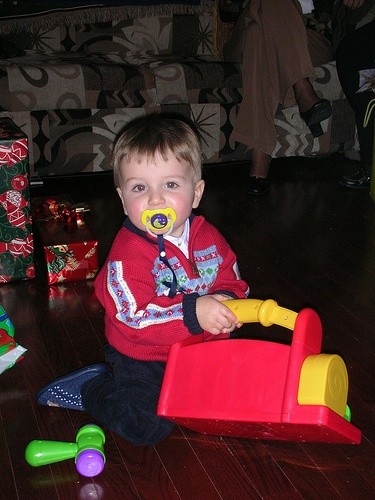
[224,0,375,195]
[38,116,249,449]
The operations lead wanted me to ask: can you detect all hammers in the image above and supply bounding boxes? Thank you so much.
[25,425,106,477]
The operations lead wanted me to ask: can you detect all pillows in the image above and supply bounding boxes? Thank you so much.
[27,11,216,58]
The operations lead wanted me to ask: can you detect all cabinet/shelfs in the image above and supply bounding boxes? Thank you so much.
[0,117,36,285]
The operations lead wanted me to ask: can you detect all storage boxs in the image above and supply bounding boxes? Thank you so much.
[32,196,99,285]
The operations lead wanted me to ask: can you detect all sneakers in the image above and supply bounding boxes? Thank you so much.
[34,363,111,412]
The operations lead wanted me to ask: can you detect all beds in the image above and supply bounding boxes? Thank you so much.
[0,0,361,189]
